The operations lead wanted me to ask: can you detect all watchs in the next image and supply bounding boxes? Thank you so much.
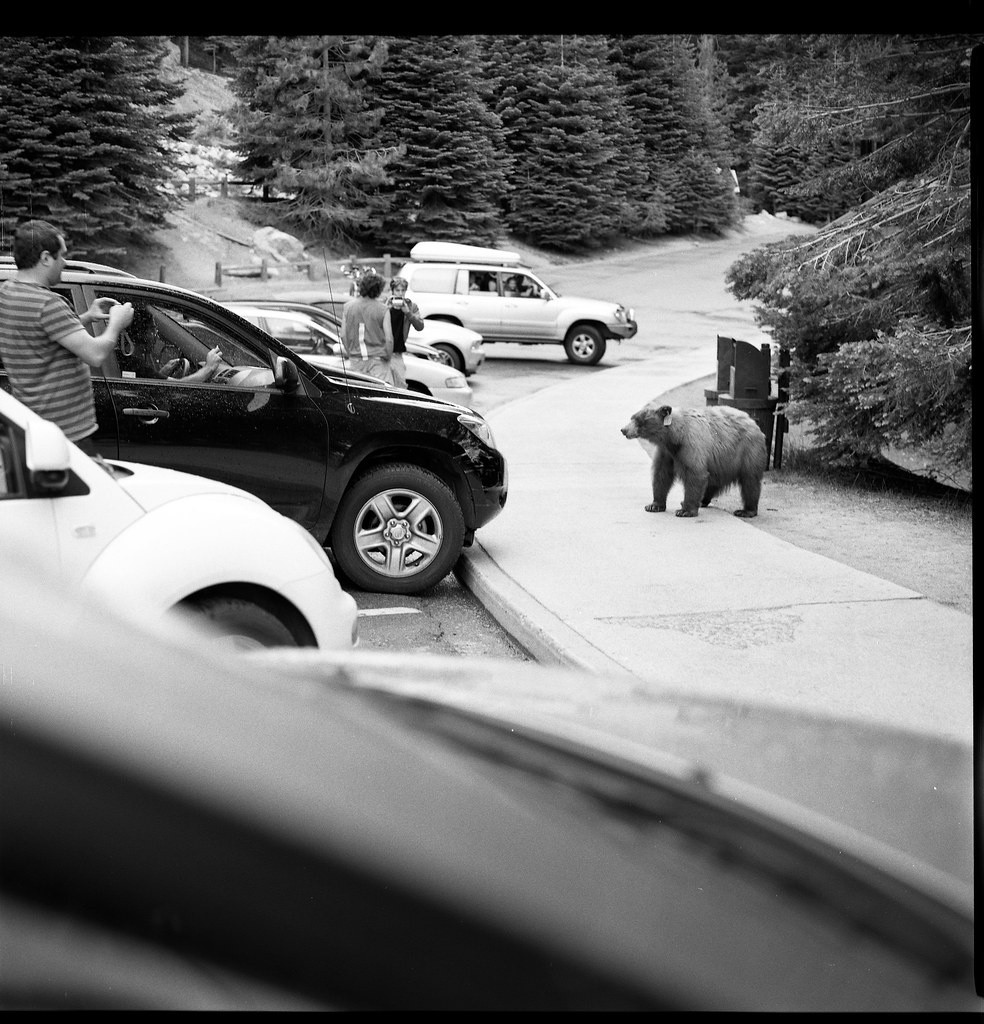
[527,286,533,288]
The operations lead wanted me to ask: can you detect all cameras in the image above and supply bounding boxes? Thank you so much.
[103,302,116,313]
[392,296,403,306]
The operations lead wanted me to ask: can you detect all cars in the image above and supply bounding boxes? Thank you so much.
[0,383,364,663]
[1,255,488,409]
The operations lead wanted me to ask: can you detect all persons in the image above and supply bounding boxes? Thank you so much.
[469,271,534,297]
[0,215,136,463]
[339,270,427,395]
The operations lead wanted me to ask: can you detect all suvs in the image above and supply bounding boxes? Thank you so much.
[1,261,507,595]
[396,240,638,366]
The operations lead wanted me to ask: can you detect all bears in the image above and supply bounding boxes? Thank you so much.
[621,401,767,518]
